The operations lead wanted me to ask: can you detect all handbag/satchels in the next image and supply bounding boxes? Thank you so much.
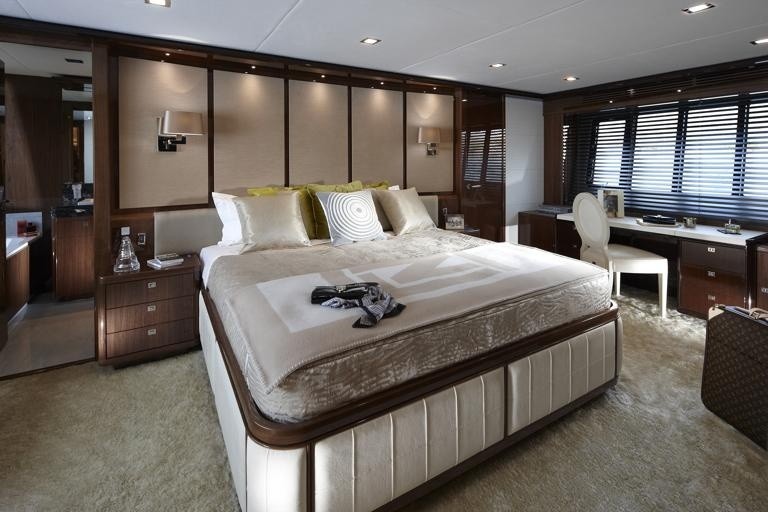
[311,286,369,304]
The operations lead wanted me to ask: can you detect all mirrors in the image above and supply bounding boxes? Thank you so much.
[461,87,506,242]
[72,110,93,186]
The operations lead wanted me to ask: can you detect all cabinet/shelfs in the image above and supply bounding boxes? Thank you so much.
[517,207,576,257]
[677,230,764,323]
[54,208,93,302]
[6,246,29,323]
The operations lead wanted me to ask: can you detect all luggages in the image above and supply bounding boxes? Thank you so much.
[701,303,768,452]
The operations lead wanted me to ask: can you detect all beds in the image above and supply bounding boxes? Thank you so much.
[200,228,619,511]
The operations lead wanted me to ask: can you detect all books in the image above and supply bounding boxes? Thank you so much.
[156,253,184,264]
[147,259,182,268]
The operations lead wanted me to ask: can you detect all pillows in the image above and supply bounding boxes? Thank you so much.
[375,188,436,237]
[231,193,311,254]
[363,181,388,191]
[247,186,316,238]
[316,190,386,246]
[307,181,362,240]
[212,192,241,246]
[373,185,399,231]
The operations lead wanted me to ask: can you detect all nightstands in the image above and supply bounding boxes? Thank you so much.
[441,227,481,237]
[94,254,203,365]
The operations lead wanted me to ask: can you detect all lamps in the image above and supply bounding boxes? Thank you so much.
[418,126,440,156]
[157,110,202,152]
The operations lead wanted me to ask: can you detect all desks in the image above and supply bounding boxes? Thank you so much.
[557,218,681,245]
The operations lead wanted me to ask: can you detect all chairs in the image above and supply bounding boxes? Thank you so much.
[573,194,668,318]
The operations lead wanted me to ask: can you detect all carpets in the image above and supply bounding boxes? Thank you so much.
[399,291,768,512]
[0,350,242,511]
